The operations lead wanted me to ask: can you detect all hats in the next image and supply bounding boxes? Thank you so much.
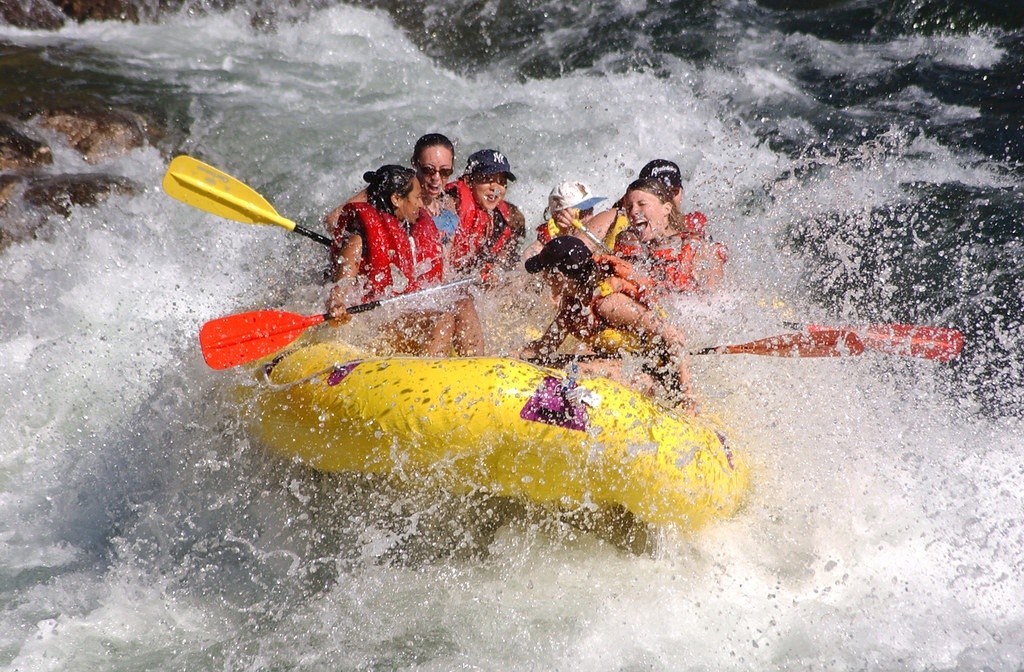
[464,149,517,184]
[639,159,683,192]
[525,236,592,276]
[549,178,608,212]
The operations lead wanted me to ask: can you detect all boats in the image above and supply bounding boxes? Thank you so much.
[241,331,751,541]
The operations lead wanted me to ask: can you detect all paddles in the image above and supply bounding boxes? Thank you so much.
[200,271,486,372]
[162,155,334,247]
[773,315,963,362]
[521,330,865,366]
[573,207,614,254]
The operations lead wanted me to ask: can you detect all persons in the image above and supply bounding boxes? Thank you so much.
[320,134,729,413]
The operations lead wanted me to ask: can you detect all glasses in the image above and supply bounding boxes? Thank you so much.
[416,160,455,177]
